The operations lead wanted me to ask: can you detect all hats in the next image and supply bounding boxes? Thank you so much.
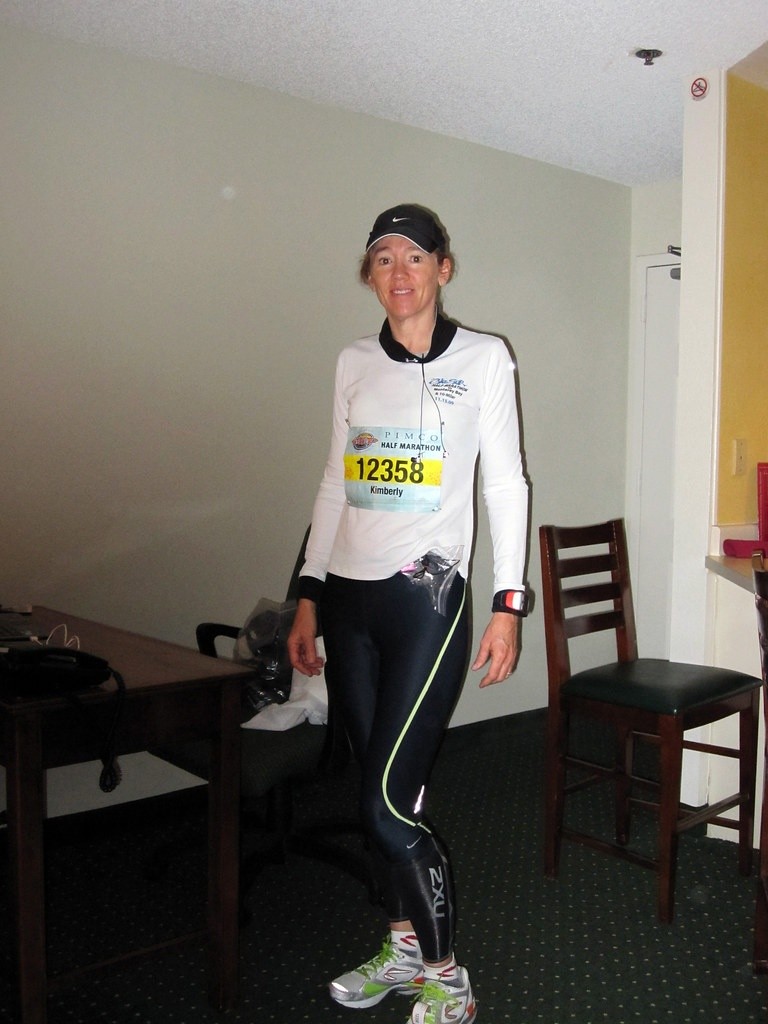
[367,204,450,255]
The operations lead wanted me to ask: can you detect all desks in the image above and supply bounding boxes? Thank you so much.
[0,604,258,1023]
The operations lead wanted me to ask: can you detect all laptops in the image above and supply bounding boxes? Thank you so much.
[0,613,52,641]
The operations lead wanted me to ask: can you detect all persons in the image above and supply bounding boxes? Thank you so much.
[286,203,533,1024]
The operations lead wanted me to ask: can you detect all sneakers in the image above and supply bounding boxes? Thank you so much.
[406,967,478,1024]
[327,932,423,1009]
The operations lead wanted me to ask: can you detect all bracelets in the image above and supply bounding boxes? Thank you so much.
[492,588,531,618]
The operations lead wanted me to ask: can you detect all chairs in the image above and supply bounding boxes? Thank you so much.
[194,621,337,865]
[537,517,763,921]
